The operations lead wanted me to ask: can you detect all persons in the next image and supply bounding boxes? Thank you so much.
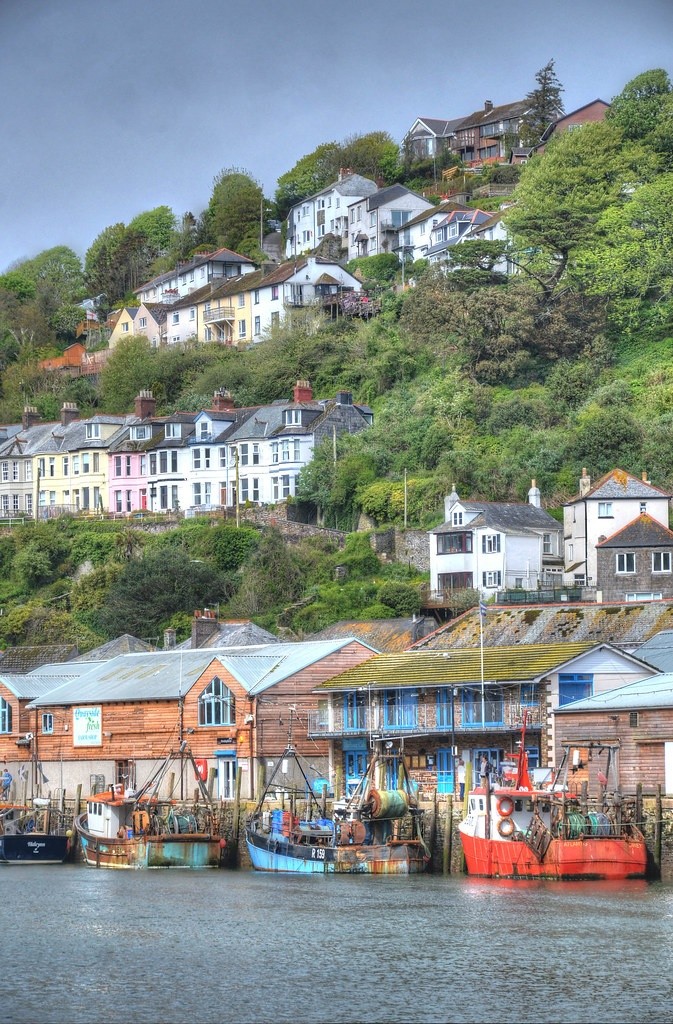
[477,756,494,789]
[0,769,13,801]
[457,759,466,801]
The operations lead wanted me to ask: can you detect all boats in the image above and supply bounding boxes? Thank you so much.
[457,712,648,880]
[244,705,431,876]
[73,649,224,870]
[0,706,69,864]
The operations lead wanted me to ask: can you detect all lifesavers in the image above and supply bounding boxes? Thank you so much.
[497,816,515,837]
[496,797,514,816]
